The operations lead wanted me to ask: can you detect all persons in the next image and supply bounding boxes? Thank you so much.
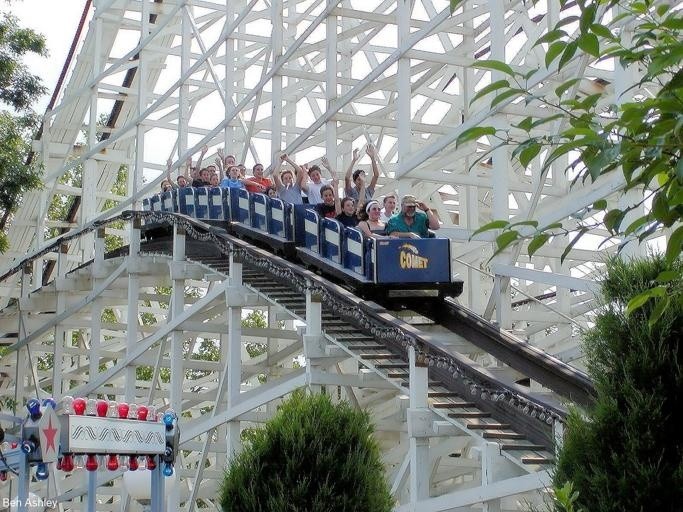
[157,141,441,242]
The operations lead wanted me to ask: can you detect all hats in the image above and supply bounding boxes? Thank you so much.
[401,195,418,208]
